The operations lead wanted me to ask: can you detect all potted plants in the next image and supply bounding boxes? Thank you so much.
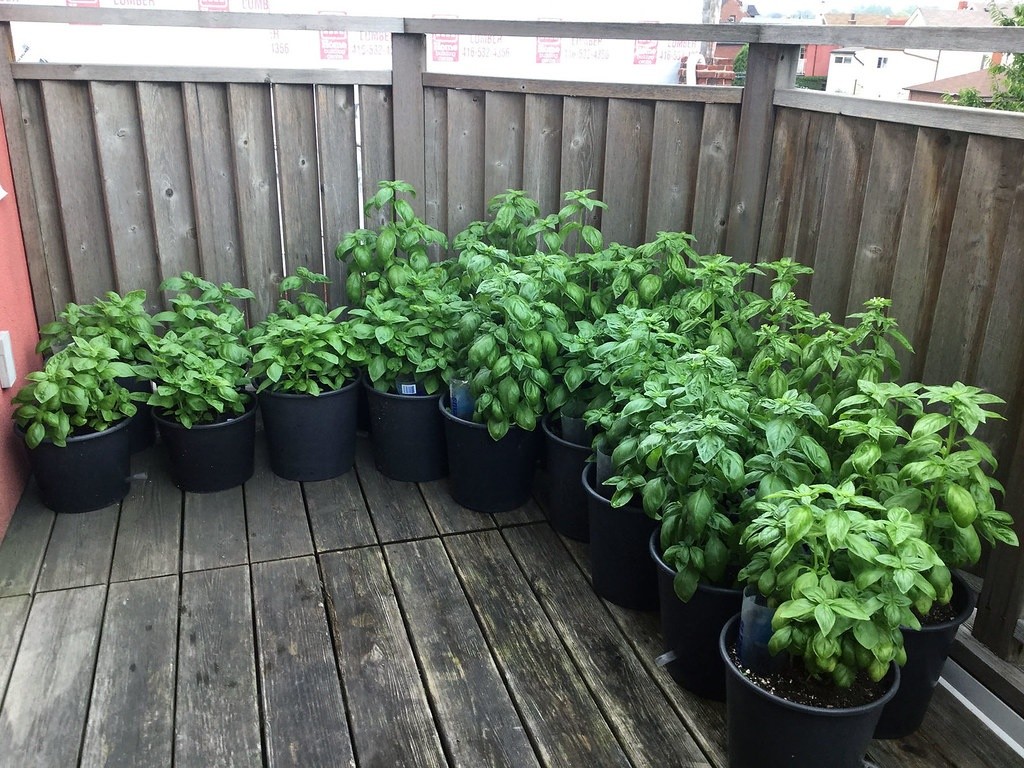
[8,177,1020,768]
